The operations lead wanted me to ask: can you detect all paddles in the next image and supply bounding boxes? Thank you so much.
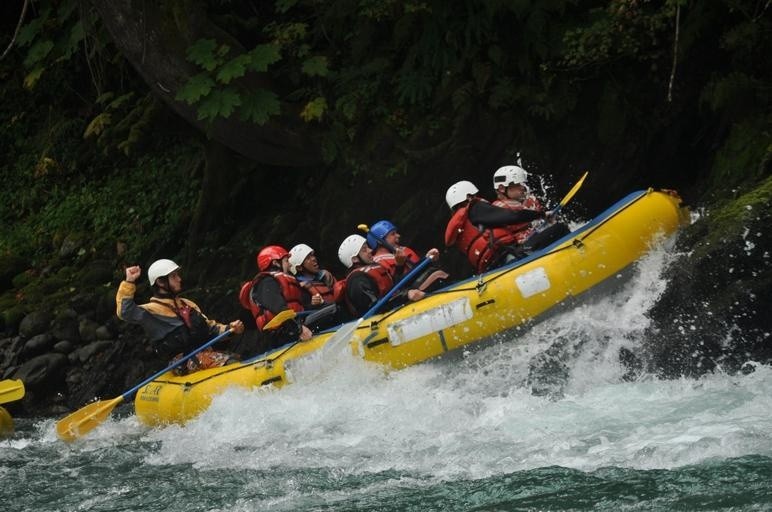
[55,327,234,442]
[522,172,587,241]
[0,377,25,403]
[322,255,432,361]
[262,310,315,329]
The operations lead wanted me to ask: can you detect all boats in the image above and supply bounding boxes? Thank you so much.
[0,406,17,441]
[131,185,707,434]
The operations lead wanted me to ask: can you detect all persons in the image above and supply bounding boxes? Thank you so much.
[337,234,441,318]
[366,221,449,294]
[287,241,349,308]
[487,164,570,252]
[114,258,246,377]
[443,180,552,272]
[249,245,323,346]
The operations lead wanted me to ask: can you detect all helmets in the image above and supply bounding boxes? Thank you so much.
[445,181,480,210]
[493,165,528,189]
[257,244,315,276]
[147,258,179,286]
[338,220,395,269]
